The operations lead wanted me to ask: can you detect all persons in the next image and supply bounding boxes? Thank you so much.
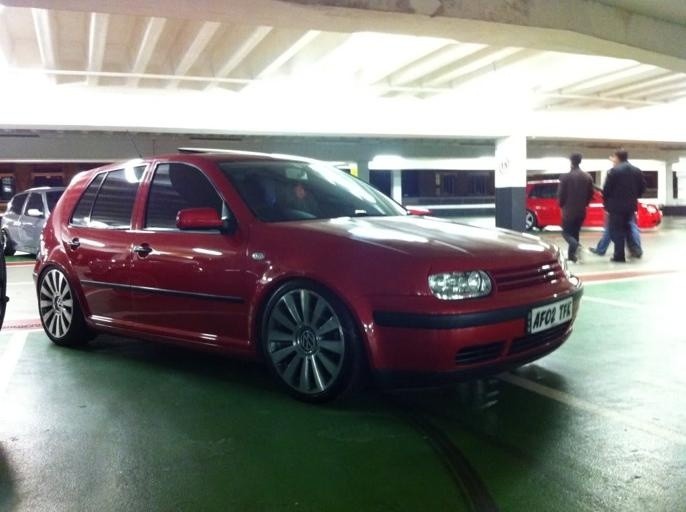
[558,152,595,263]
[602,147,647,261]
[590,173,647,257]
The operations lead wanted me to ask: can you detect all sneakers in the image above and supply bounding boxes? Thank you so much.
[573,243,640,261]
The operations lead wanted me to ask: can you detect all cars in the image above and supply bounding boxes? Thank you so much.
[525,178,663,233]
[0,186,68,256]
[30,145,585,407]
